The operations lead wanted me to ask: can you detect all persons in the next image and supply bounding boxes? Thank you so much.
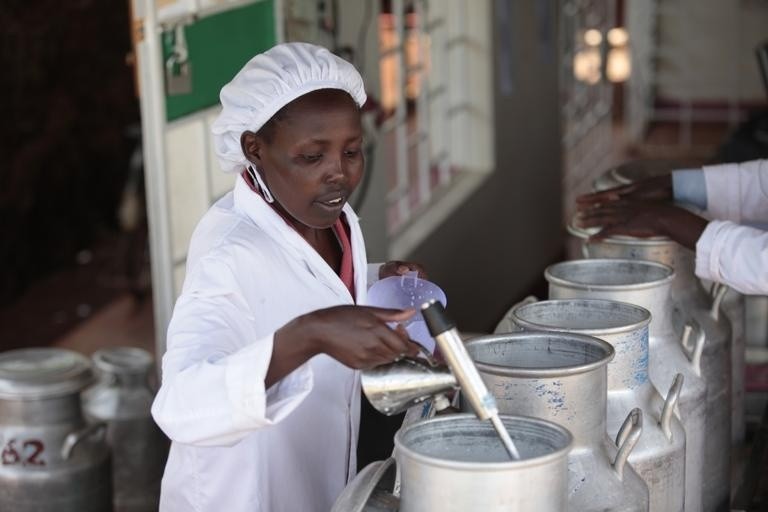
[149,42,424,511]
[573,158,768,296]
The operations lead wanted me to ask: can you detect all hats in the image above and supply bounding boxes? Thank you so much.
[208,42,368,204]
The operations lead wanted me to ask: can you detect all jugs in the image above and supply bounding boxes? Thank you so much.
[362,336,464,418]
[369,269,449,351]
[460,333,650,512]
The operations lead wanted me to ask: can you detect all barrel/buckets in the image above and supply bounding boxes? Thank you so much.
[566,201,735,512]
[78,343,167,512]
[392,414,575,511]
[507,301,689,512]
[544,257,709,512]
[1,345,110,511]
